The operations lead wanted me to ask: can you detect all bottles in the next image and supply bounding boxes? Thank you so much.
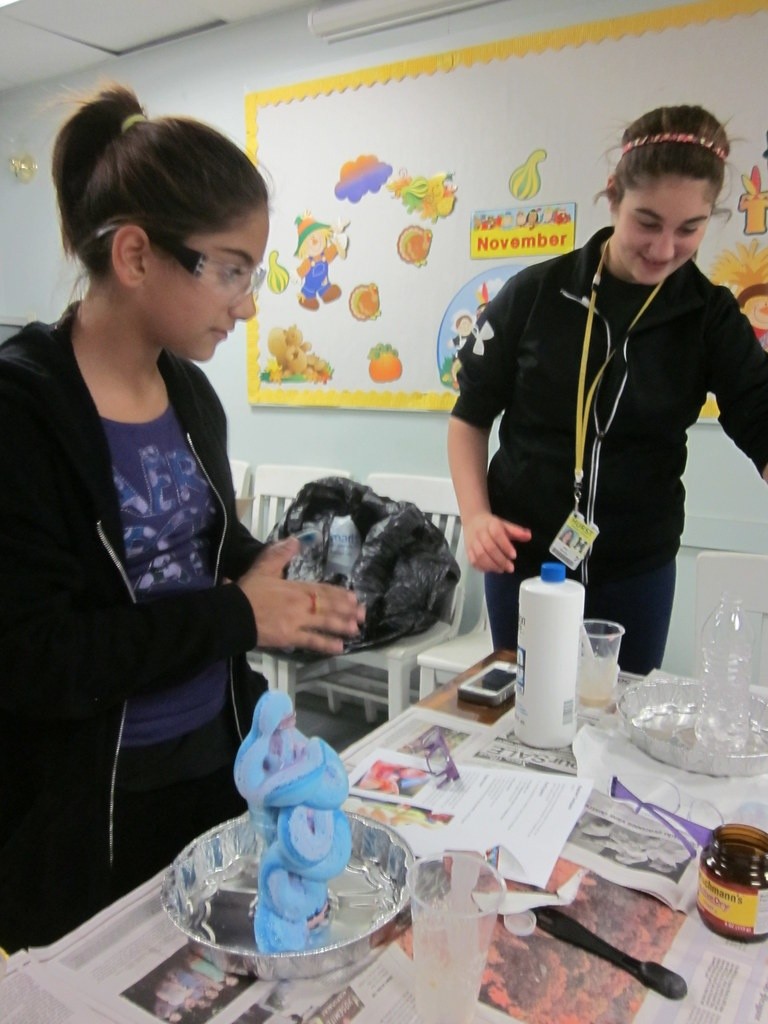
[693,588,754,756]
[512,562,586,750]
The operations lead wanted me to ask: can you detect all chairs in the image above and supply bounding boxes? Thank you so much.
[694,552,768,689]
[416,594,497,703]
[246,464,350,692]
[228,459,252,514]
[277,472,471,725]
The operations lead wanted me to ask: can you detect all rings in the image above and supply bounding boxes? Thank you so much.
[308,590,319,613]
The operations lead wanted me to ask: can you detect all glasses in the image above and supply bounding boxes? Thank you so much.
[421,725,460,789]
[95,223,268,307]
[610,773,721,858]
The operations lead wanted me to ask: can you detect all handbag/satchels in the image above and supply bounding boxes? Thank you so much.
[264,476,461,664]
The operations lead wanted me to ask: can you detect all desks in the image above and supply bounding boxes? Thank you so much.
[0,648,768,1023]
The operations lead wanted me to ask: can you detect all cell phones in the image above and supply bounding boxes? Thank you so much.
[457,661,519,707]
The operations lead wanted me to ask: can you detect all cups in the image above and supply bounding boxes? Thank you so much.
[578,617,626,709]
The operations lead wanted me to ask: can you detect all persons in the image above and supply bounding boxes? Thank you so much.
[0,83,366,956]
[447,104,768,674]
[559,530,574,546]
